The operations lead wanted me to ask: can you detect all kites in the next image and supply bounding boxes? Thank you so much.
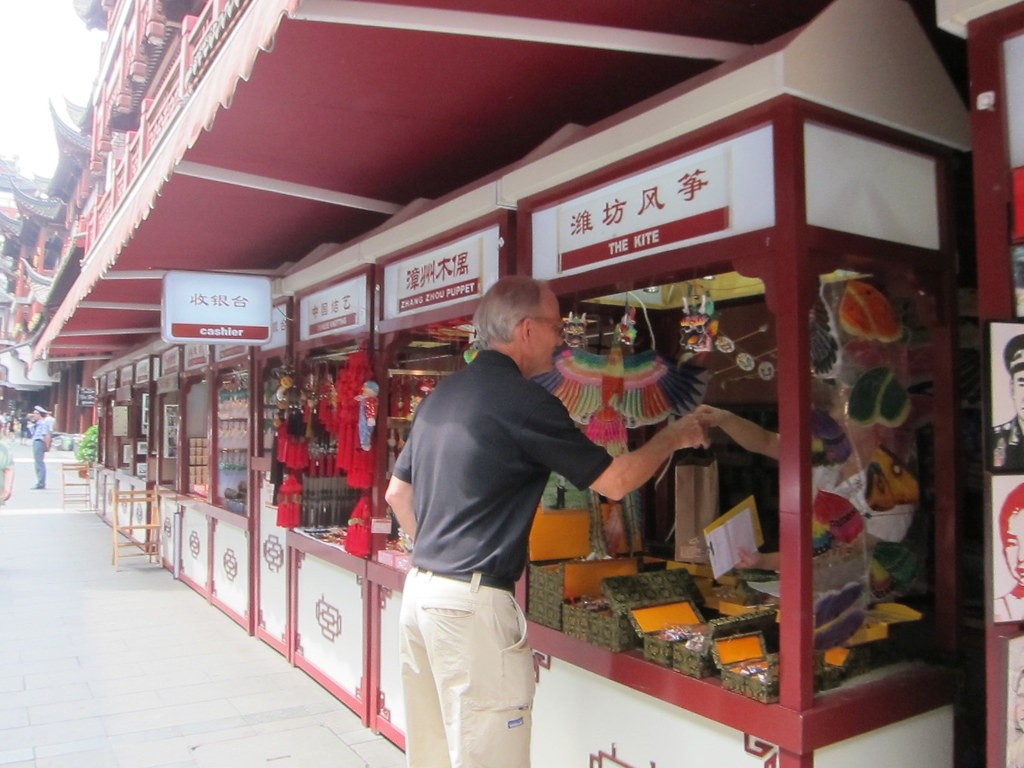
[529,308,709,561]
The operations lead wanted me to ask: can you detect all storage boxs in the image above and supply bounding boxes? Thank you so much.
[527,507,922,705]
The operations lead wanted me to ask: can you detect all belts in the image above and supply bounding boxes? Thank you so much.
[419,567,515,592]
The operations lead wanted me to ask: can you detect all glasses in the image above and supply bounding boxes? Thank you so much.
[518,315,565,332]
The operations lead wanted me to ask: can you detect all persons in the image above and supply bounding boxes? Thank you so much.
[0,420,14,505]
[352,380,381,452]
[30,405,53,489]
[1,409,56,448]
[384,272,712,768]
[693,379,931,616]
[553,475,567,510]
[274,374,296,421]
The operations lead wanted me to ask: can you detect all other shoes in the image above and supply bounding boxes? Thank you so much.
[31,486,44,489]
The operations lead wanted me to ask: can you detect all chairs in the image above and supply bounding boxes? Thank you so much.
[62,460,92,511]
[112,479,162,571]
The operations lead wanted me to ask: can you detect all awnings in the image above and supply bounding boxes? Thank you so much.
[22,1,836,359]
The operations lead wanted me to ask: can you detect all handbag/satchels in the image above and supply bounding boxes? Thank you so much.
[674,447,718,564]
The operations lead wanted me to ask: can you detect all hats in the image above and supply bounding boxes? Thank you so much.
[1004,334,1024,374]
